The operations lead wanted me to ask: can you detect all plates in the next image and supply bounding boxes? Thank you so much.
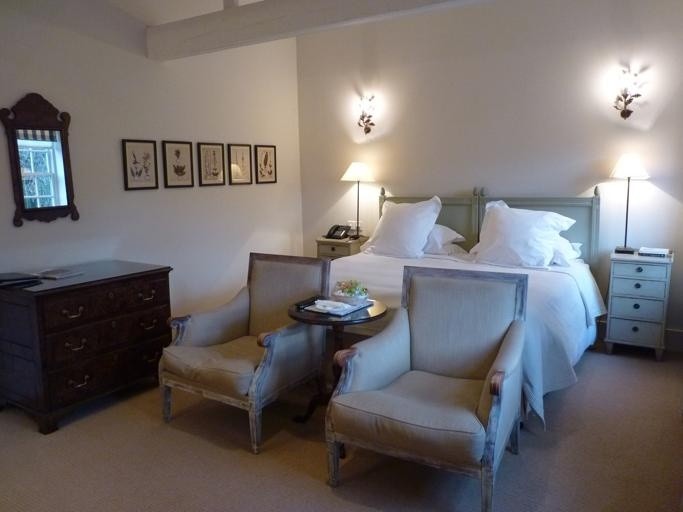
[0,260,174,434]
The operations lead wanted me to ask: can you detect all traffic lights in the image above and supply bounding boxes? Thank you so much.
[361,196,584,266]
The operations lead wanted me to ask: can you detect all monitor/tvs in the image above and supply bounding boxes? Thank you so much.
[301,295,375,316]
[21,264,85,279]
[637,246,669,258]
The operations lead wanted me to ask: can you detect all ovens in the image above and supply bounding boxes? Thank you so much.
[327,187,607,435]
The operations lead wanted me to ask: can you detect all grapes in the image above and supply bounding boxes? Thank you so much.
[604,253,674,362]
[316,237,363,258]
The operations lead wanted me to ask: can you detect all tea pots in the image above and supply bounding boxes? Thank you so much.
[0,94,82,226]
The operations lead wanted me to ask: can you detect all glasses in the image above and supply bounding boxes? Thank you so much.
[610,155,651,255]
[594,61,656,120]
[340,162,375,239]
[354,85,383,137]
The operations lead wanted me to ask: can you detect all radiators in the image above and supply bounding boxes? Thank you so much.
[295,296,321,309]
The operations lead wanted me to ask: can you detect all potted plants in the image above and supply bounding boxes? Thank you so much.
[228,143,253,184]
[162,140,194,188]
[122,139,158,190]
[198,142,225,186]
[254,144,277,184]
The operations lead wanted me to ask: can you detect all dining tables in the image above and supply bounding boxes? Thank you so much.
[336,277,368,305]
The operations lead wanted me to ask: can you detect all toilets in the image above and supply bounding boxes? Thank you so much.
[326,225,351,239]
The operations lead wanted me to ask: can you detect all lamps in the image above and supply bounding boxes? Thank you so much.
[324,265,530,511]
[158,252,331,454]
